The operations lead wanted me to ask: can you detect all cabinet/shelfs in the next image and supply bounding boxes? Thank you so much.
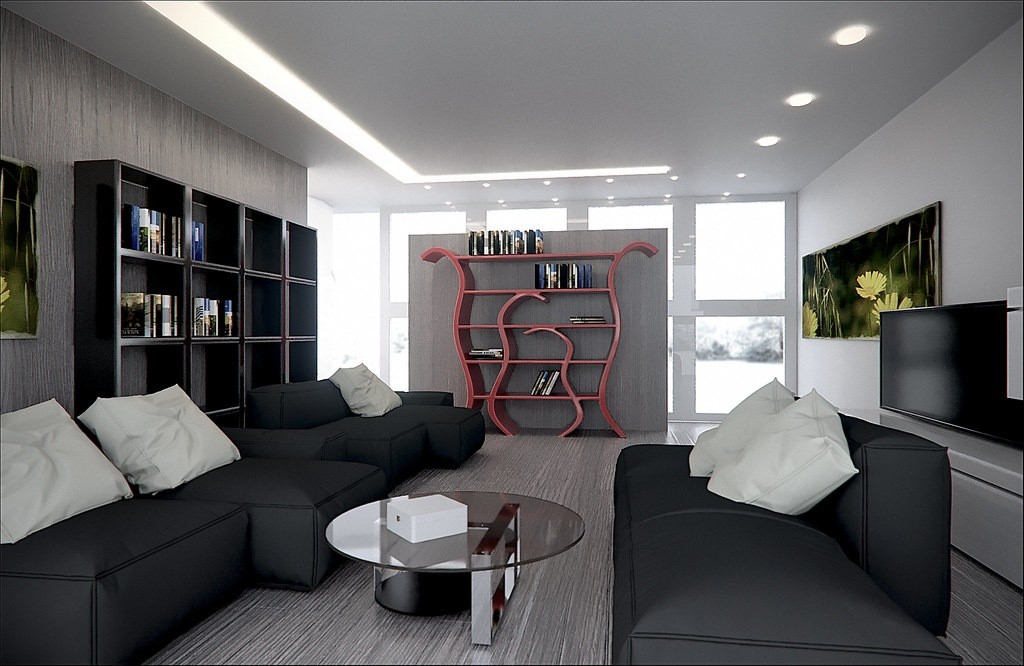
[420,241,660,438]
[72,158,318,425]
[879,411,1023,590]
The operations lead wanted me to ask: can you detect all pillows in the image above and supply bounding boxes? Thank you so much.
[707,387,859,516]
[75,383,241,495]
[689,378,795,478]
[0,398,134,547]
[329,362,403,418]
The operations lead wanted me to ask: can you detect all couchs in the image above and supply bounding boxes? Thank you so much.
[612,411,962,666]
[0,380,484,666]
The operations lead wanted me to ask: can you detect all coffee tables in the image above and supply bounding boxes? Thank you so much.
[326,492,588,644]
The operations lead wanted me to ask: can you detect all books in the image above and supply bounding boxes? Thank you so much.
[192,221,205,262]
[530,370,561,396]
[465,348,504,360]
[534,263,593,289]
[123,202,182,258]
[467,229,544,256]
[121,292,178,338]
[192,297,233,337]
[570,316,606,324]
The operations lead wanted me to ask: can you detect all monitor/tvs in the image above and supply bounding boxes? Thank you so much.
[878,299,1011,443]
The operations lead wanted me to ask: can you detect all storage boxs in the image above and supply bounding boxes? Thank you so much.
[386,494,469,544]
